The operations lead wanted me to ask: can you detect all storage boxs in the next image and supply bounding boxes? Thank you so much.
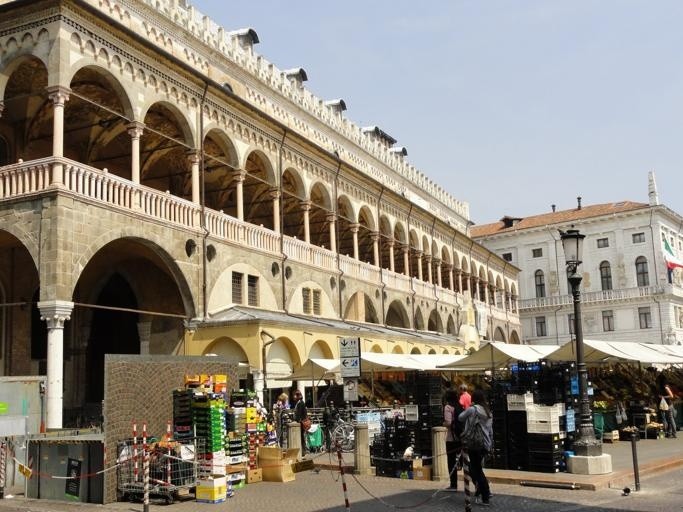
[170,373,299,503]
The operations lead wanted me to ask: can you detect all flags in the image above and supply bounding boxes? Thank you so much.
[663,237,683,269]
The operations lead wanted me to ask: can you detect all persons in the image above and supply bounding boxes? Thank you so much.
[255,368,682,459]
[442,390,476,492]
[459,391,494,506]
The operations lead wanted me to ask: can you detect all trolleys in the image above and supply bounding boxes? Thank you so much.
[114,432,197,504]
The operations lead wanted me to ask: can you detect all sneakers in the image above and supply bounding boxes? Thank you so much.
[445,486,456,492]
[476,499,489,506]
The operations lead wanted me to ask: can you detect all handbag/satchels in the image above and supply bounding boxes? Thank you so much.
[460,424,482,454]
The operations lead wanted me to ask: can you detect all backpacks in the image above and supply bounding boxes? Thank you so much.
[451,403,465,437]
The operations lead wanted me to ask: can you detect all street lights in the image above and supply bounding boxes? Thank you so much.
[555,221,603,456]
